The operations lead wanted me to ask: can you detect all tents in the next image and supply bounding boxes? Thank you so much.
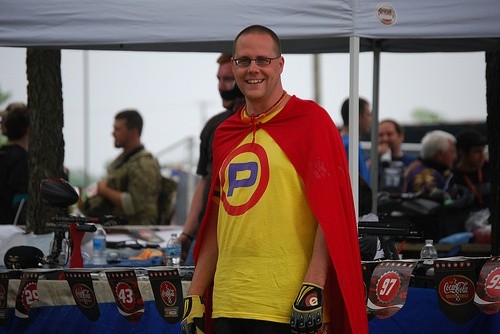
[0,1,499,232]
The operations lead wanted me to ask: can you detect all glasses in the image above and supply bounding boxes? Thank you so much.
[232,55,281,67]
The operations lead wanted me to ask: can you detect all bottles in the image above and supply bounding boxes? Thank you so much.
[380,147,392,168]
[165,233,182,268]
[420,239,438,276]
[92,224,107,265]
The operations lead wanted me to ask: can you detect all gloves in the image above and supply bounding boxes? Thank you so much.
[181,295,206,334]
[289,282,325,334]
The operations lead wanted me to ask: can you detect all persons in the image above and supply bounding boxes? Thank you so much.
[162,29,367,334]
[340,97,488,195]
[97,110,161,225]
[0,103,70,225]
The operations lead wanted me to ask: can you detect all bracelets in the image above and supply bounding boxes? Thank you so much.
[181,232,194,241]
